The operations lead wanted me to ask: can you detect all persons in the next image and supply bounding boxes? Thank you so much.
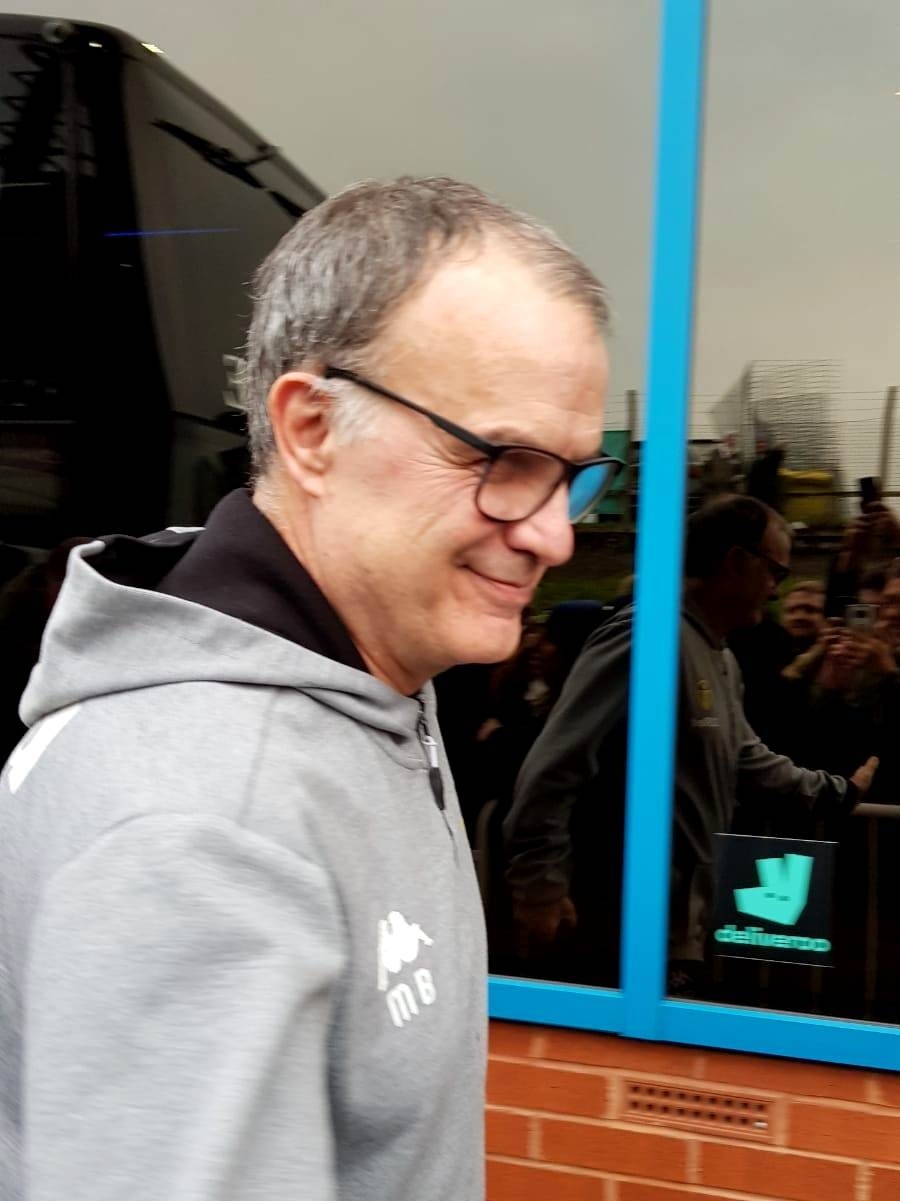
[433,596,601,973]
[500,494,878,996]
[708,432,900,809]
[0,173,612,1200]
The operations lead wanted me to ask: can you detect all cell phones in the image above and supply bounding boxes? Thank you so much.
[860,475,882,504]
[845,604,878,634]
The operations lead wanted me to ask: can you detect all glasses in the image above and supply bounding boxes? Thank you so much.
[321,364,631,530]
[738,536,793,586]
